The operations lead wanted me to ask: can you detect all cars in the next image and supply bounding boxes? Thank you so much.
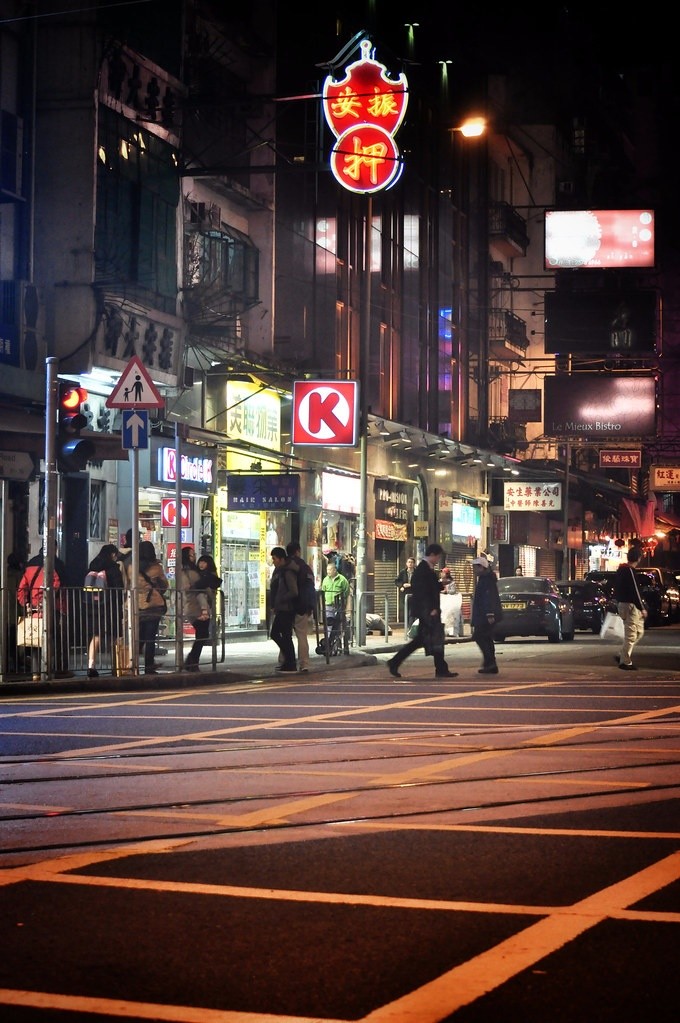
[584,567,680,626]
[493,577,575,644]
[555,580,612,633]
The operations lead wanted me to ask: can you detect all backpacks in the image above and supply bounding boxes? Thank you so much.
[83,570,107,604]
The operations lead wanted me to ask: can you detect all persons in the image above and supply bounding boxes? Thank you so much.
[612,548,648,671]
[321,563,350,631]
[7,539,73,680]
[515,566,523,576]
[584,572,588,581]
[86,529,170,677]
[394,558,457,595]
[386,544,459,678]
[269,542,315,674]
[470,558,504,674]
[182,546,223,673]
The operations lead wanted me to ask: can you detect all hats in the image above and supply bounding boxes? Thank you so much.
[468,557,488,568]
[440,568,450,573]
[324,549,337,555]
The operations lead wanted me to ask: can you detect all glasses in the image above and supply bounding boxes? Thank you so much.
[189,553,198,557]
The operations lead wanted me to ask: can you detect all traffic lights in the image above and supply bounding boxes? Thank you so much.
[56,382,95,472]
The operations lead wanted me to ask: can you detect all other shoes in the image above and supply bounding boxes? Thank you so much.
[436,670,458,677]
[388,660,401,676]
[196,614,210,622]
[276,665,297,673]
[33,676,40,681]
[87,668,99,677]
[619,663,637,670]
[479,666,498,673]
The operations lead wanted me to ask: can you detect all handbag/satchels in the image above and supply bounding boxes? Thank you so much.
[601,612,625,642]
[424,616,444,655]
[17,617,43,647]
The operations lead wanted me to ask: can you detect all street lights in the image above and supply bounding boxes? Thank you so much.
[353,118,487,635]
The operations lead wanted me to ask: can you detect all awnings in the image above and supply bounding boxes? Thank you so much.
[653,512,680,530]
[366,411,631,521]
